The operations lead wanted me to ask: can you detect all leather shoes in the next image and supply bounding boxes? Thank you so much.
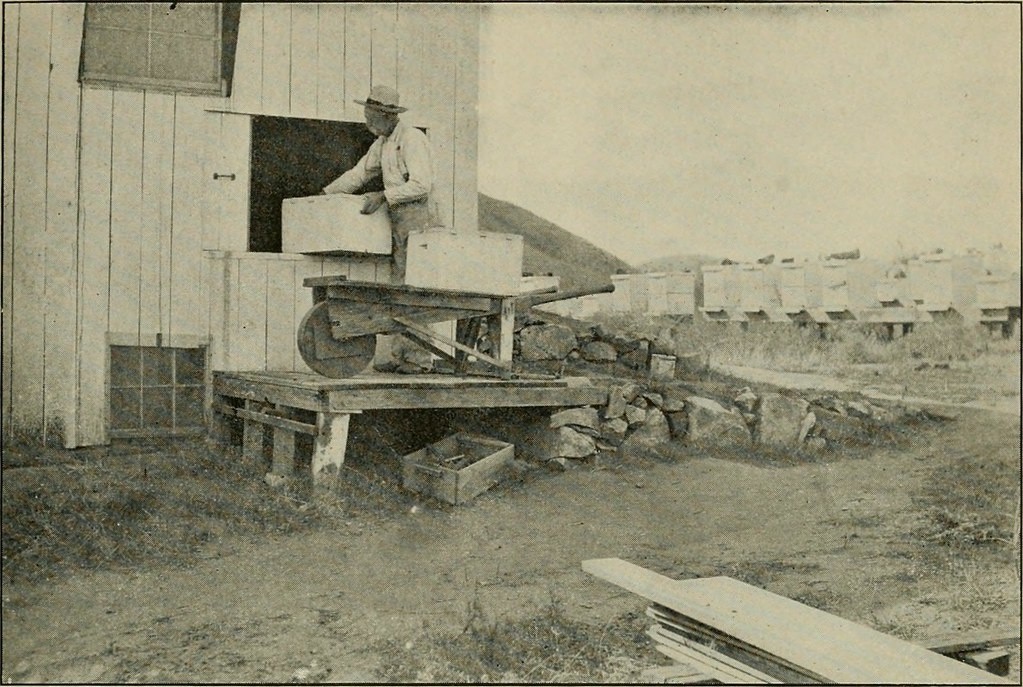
[372,361,426,375]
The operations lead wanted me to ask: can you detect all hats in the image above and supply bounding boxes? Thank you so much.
[350,83,407,113]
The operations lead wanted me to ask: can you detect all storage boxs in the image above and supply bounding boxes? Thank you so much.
[400,431,515,506]
[404,228,525,299]
[280,194,393,257]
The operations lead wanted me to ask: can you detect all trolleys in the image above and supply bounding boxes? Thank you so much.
[297,275,616,380]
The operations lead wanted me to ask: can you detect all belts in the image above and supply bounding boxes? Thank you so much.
[390,198,426,207]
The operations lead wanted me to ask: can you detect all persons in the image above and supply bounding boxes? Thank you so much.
[318,82,442,377]
